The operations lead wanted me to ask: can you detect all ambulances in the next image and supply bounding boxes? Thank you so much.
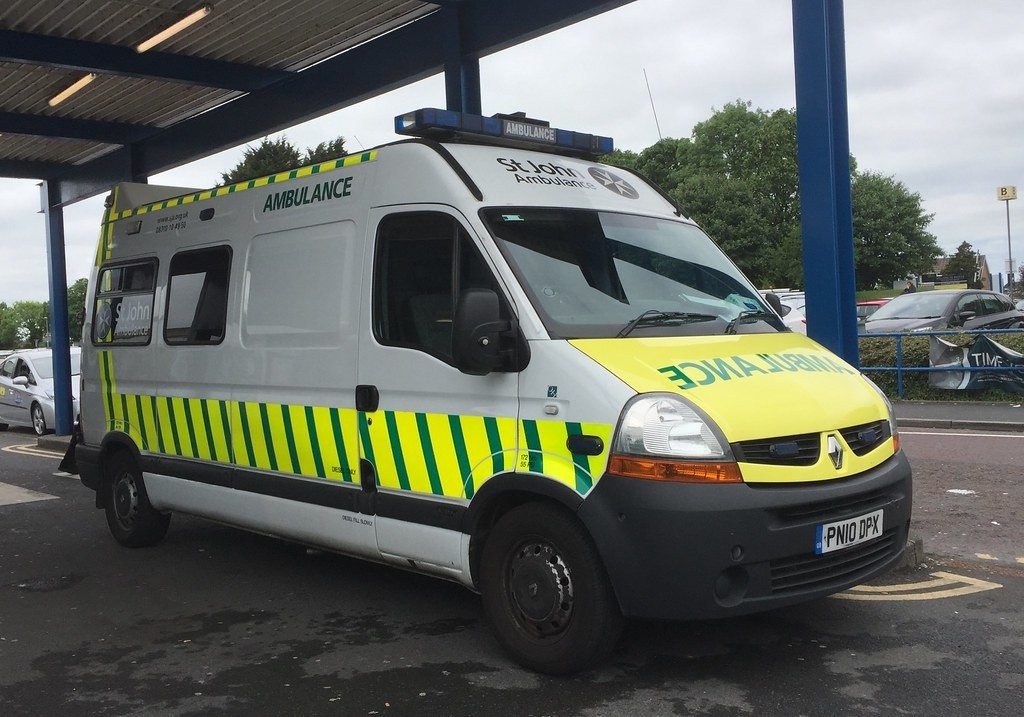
[48,109,912,669]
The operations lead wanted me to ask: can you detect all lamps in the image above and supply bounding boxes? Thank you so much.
[133,3,214,54]
[48,73,96,107]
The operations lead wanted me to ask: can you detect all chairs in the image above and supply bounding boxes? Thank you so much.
[384,252,460,353]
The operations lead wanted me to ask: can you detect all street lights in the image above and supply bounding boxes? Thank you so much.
[44,316,49,348]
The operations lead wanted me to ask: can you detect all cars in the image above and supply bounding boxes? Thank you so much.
[759,288,806,336]
[0,348,82,435]
[857,289,1024,330]
[857,299,889,320]
